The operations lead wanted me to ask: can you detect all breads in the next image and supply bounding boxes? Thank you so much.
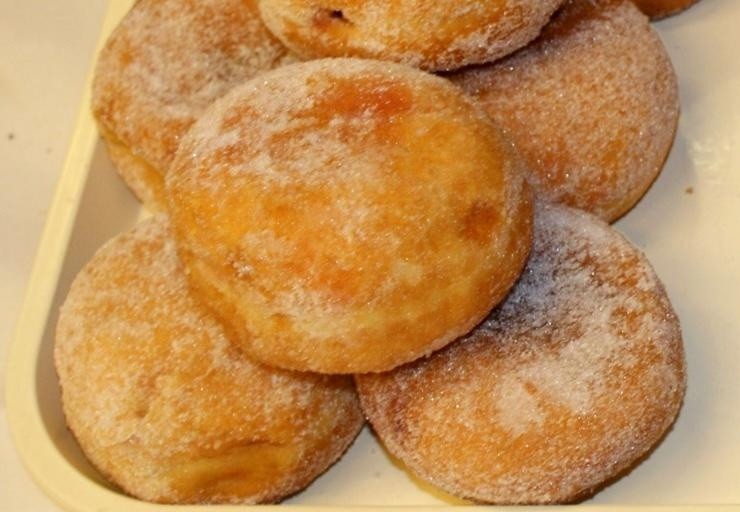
[161,60,533,375]
[354,202,685,504]
[634,1,697,20]
[257,0,563,73]
[437,2,680,224]
[90,0,304,211]
[54,211,364,497]
